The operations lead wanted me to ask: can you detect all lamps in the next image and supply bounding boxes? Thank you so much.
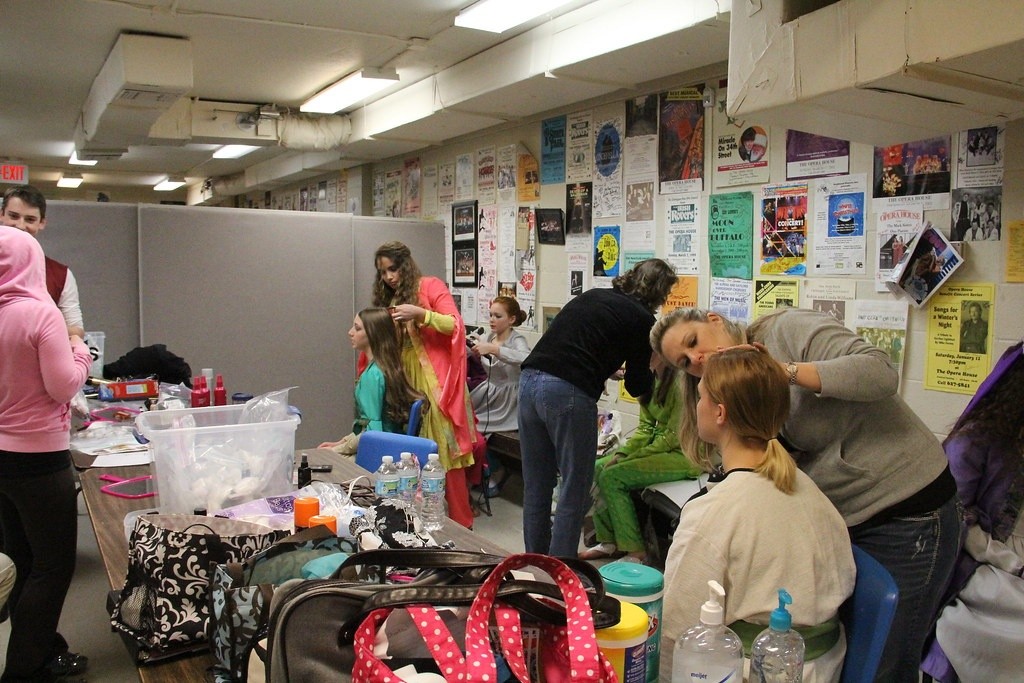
[300,66,400,114]
[56,175,83,188]
[453,0,575,34]
[153,178,186,191]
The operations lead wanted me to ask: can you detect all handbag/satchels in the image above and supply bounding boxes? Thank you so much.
[109,512,620,683]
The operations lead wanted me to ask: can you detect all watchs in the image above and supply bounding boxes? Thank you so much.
[787,360,798,384]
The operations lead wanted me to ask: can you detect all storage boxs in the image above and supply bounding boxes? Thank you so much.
[84,331,105,378]
[132,404,302,516]
[99,379,159,401]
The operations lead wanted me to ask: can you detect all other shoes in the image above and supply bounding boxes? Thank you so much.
[49,650,86,675]
[485,467,511,497]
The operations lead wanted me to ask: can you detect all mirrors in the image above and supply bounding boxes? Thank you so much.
[98,474,158,499]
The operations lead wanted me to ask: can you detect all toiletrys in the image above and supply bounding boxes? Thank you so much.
[748,587,806,683]
[671,580,745,683]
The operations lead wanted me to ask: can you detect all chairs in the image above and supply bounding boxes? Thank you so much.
[407,400,422,436]
[839,545,900,683]
[355,432,439,473]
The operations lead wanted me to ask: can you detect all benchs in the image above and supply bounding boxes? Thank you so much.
[480,431,595,546]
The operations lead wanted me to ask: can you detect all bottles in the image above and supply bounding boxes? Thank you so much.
[395,452,419,510]
[149,400,158,411]
[598,561,665,683]
[191,368,214,407]
[294,497,319,533]
[298,453,311,489]
[308,514,337,539]
[232,391,254,405]
[594,601,648,683]
[375,456,400,499]
[214,375,227,406]
[420,453,445,531]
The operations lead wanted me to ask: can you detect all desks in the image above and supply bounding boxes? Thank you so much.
[69,399,559,683]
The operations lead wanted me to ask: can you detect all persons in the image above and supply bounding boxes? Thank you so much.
[0,184,86,338]
[913,147,947,174]
[0,223,94,683]
[892,236,907,265]
[915,244,941,275]
[922,342,1024,683]
[739,127,767,162]
[317,306,430,464]
[953,193,1000,241]
[470,296,532,498]
[649,308,960,683]
[660,350,857,683]
[577,361,724,563]
[960,303,988,354]
[517,258,680,558]
[969,132,995,157]
[358,239,477,532]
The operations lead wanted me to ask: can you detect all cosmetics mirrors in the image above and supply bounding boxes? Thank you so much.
[84,407,140,427]
[100,474,155,499]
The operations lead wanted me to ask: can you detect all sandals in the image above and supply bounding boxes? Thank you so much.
[577,543,624,559]
[614,555,651,565]
